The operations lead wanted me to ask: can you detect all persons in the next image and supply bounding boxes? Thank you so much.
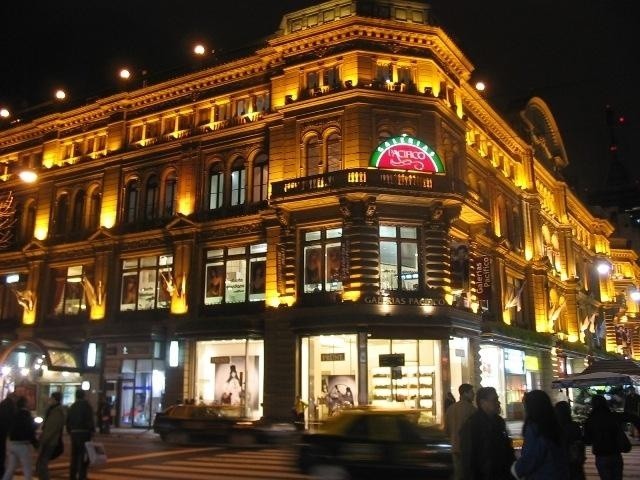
[36,392,66,479]
[293,396,308,429]
[581,390,634,479]
[550,400,586,479]
[0,393,17,479]
[97,399,112,434]
[510,389,567,479]
[623,385,640,442]
[176,391,233,407]
[3,396,39,479]
[443,384,478,479]
[459,388,518,477]
[66,388,96,479]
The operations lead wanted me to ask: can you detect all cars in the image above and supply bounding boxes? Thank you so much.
[152,403,297,450]
[296,410,455,480]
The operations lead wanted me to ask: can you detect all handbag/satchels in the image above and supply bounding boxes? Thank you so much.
[84,436,108,468]
[49,436,64,460]
[613,413,634,453]
